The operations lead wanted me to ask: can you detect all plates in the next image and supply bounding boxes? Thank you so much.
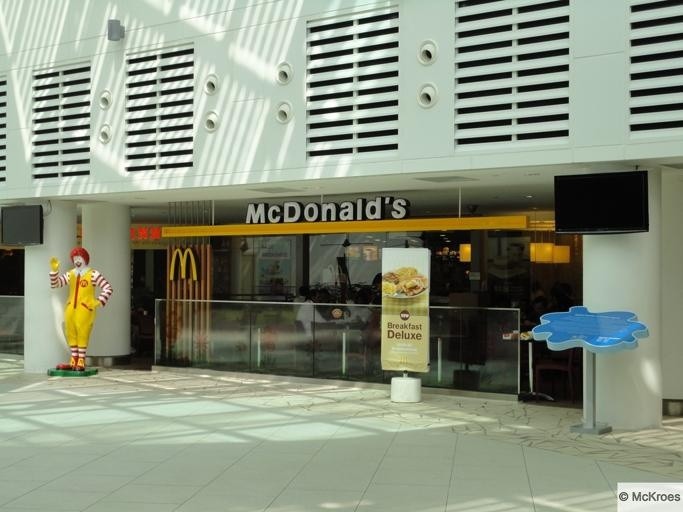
[384,286,428,300]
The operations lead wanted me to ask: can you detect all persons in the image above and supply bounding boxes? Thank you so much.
[292,273,583,376]
[49,247,113,371]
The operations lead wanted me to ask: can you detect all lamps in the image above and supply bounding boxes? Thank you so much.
[459,226,472,263]
[108,19,124,41]
[529,206,571,266]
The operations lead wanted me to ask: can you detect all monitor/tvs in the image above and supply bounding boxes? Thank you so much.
[1,205,43,244]
[553,171,649,235]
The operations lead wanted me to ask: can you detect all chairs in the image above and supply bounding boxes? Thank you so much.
[255,301,579,404]
